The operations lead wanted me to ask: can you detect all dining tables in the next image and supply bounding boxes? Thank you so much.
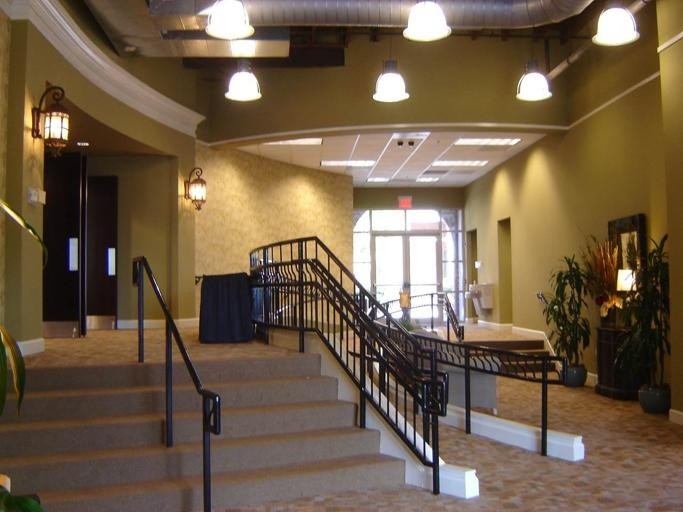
[617,231,668,412]
[542,255,596,385]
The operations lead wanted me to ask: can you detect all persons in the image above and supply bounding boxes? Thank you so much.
[398,282,412,326]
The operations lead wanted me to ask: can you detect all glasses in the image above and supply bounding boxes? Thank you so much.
[182,167,209,211]
[31,84,72,158]
[401,0,451,45]
[588,1,641,45]
[203,0,257,38]
[221,57,262,104]
[512,32,552,103]
[370,33,411,102]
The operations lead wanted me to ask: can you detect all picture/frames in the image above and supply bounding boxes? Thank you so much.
[198,272,263,344]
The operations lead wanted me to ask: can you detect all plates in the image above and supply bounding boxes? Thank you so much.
[592,326,642,402]
